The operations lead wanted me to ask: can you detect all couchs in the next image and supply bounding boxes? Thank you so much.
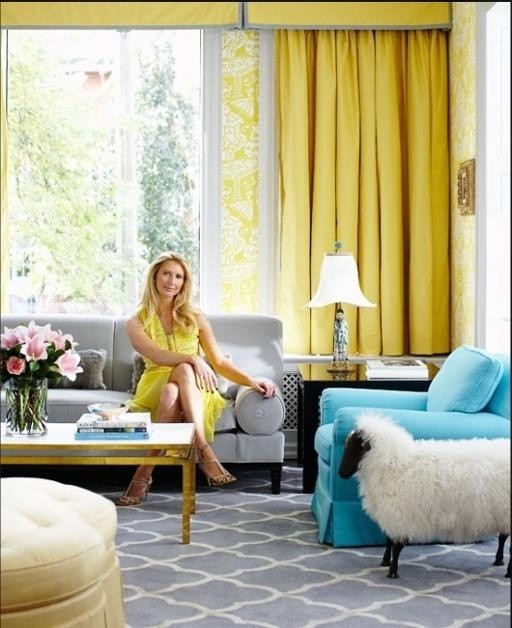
[311,345,511,548]
[0,313,285,493]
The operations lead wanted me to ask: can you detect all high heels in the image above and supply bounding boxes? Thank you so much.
[113,474,154,507]
[195,441,237,486]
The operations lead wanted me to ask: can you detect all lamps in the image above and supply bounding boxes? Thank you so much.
[305,253,378,373]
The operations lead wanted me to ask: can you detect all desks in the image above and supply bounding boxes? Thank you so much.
[1,423,198,548]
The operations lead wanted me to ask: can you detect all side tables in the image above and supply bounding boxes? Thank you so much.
[297,361,440,493]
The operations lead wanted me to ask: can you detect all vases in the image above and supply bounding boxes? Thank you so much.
[6,380,48,437]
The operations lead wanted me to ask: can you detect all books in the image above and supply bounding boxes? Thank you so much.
[365,359,429,380]
[74,411,151,441]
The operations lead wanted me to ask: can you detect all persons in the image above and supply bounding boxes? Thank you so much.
[113,250,277,507]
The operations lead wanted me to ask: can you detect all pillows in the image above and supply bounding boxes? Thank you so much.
[128,354,231,395]
[427,345,503,413]
[47,350,108,389]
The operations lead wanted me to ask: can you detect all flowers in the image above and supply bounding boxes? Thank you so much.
[0,321,84,436]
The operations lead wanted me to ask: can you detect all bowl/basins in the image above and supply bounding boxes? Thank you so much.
[87,403,130,419]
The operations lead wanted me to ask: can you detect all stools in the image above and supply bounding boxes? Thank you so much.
[1,477,125,628]
